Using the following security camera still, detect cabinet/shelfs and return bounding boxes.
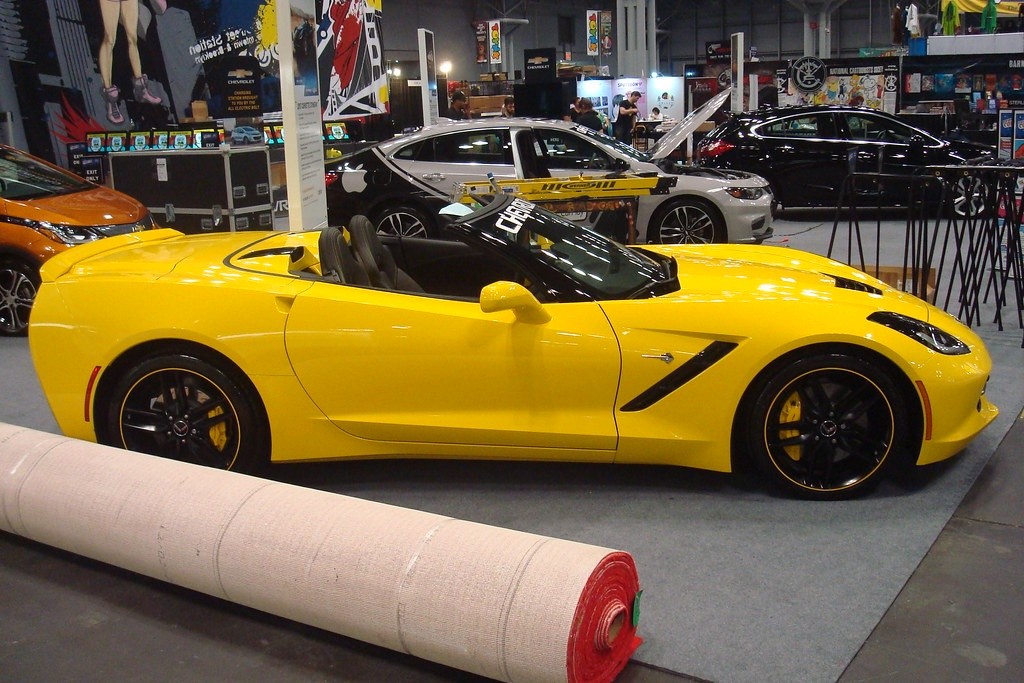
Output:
[897,100,956,115]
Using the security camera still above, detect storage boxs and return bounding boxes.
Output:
[558,65,596,79]
[479,73,493,82]
[849,265,935,309]
[148,207,274,235]
[494,73,506,81]
[463,95,515,119]
[109,145,274,215]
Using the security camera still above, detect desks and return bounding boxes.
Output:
[610,120,664,145]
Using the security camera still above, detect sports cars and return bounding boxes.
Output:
[28,192,999,501]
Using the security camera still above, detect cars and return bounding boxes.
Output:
[325,117,778,245]
[231,126,262,145]
[692,103,998,220]
[0,143,162,337]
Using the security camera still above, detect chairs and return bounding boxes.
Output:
[635,124,648,152]
[318,227,373,288]
[816,113,855,140]
[350,215,426,293]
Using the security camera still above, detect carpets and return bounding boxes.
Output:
[1,215,1023,683]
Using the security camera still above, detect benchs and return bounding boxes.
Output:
[413,138,470,163]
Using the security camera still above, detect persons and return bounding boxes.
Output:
[443,90,474,121]
[495,96,514,118]
[614,90,642,146]
[563,96,604,134]
[647,105,664,122]
